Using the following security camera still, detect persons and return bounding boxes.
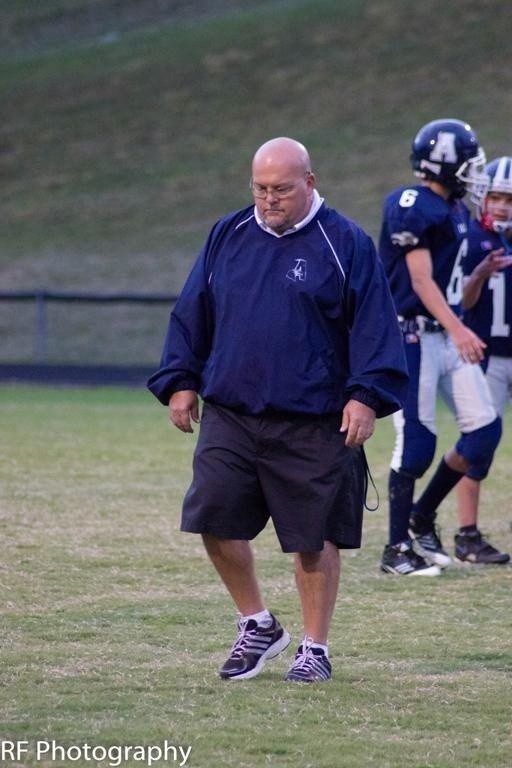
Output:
[145,135,412,686]
[377,118,503,582]
[452,154,511,571]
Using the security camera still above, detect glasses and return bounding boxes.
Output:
[249,171,311,200]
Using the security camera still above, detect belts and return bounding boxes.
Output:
[399,319,444,332]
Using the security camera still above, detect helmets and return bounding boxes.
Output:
[475,157,512,234]
[409,118,490,208]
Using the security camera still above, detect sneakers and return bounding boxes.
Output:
[454,530,510,563]
[284,635,333,683]
[407,503,452,568]
[219,612,292,681]
[380,538,442,576]
